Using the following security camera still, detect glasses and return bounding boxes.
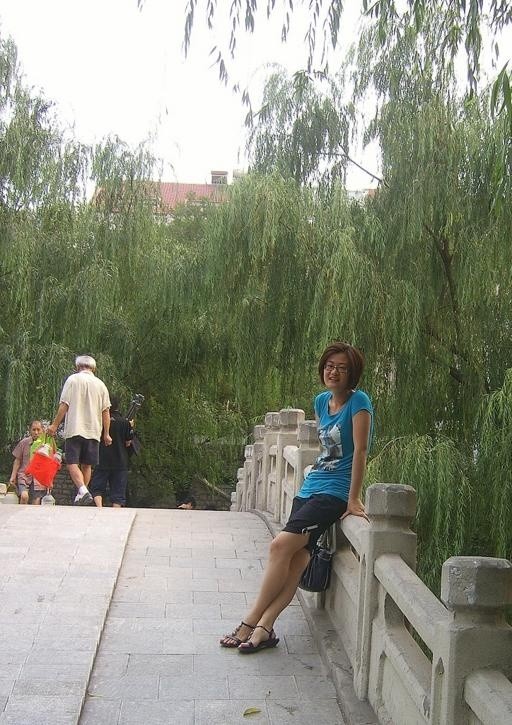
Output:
[324,362,351,375]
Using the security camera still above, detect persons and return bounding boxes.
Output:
[89,396,133,507]
[219,342,374,654]
[9,420,53,505]
[45,355,113,506]
[176,496,196,510]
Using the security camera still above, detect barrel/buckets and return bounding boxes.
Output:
[3,484,19,504]
[41,493,56,506]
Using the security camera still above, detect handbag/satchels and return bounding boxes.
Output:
[298,519,336,593]
[41,488,54,507]
[0,484,19,504]
[24,432,64,486]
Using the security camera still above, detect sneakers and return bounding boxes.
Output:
[74,492,93,506]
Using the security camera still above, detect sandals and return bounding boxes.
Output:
[219,620,253,647]
[236,625,279,652]
